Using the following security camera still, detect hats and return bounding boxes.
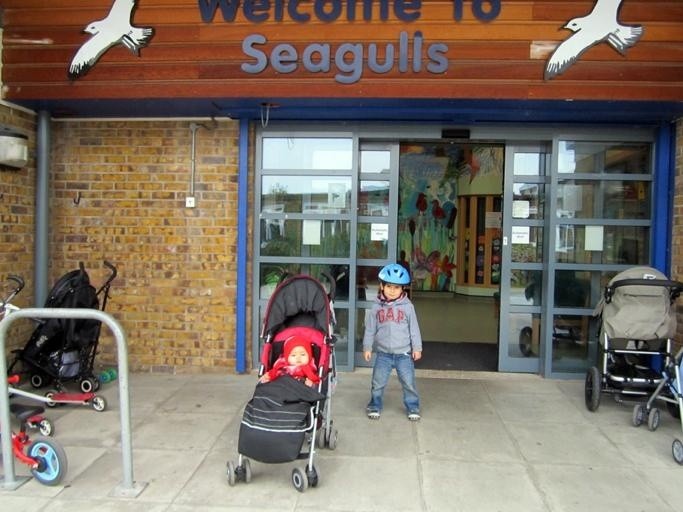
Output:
[284,336,312,363]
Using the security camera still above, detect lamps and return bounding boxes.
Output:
[0,128,28,172]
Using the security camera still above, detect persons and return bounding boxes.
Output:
[258,335,319,387]
[362,264,422,422]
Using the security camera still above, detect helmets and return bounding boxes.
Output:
[378,264,411,286]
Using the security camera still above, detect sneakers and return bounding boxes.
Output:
[407,410,420,420]
[367,411,380,419]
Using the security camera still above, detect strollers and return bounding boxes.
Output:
[584,266,683,468]
[227,270,339,492]
[6,258,117,408]
